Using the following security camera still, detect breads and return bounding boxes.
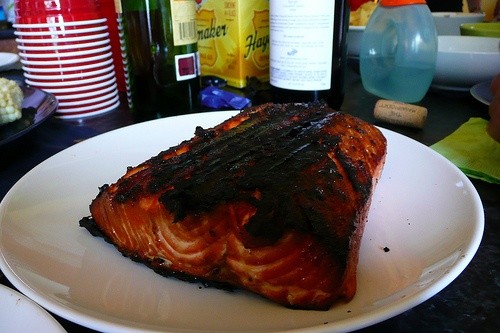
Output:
[0,78,22,124]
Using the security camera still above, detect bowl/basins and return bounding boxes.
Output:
[430,35,500,92]
[430,11,486,36]
[350,25,367,59]
[460,23,500,38]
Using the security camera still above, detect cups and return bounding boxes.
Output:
[13,0,121,121]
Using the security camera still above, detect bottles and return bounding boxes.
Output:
[268,0,349,109]
[123,0,201,120]
[360,0,438,103]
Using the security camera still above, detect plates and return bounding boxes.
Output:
[470,82,492,105]
[0,51,20,71]
[0,110,485,333]
[0,284,67,333]
[0,85,59,145]
